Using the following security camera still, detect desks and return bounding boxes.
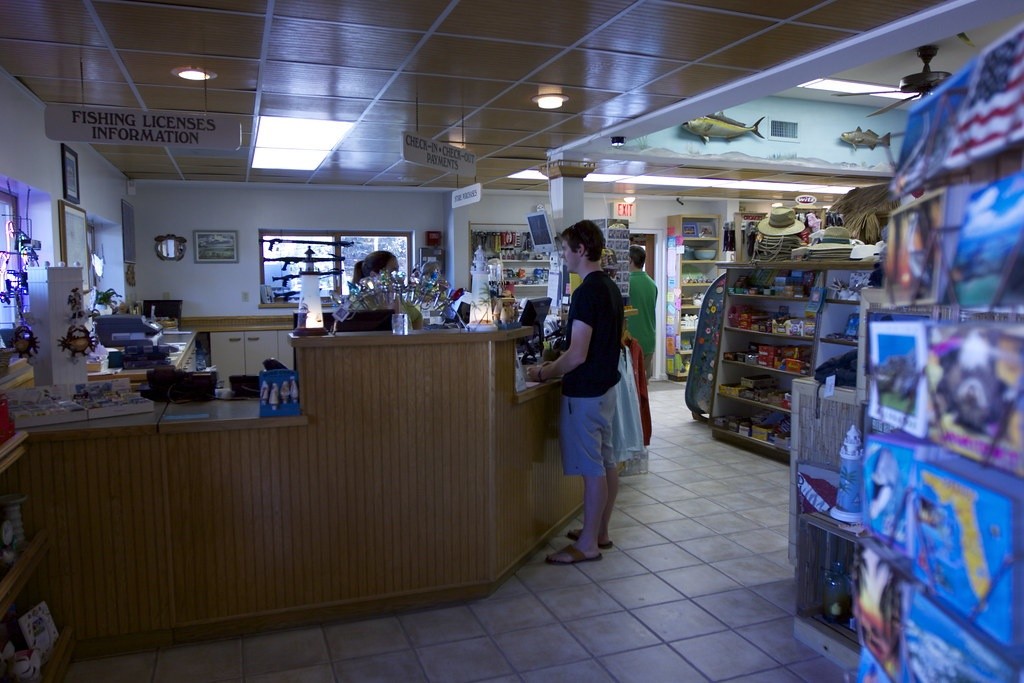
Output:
[17,331,196,658]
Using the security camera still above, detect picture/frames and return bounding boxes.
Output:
[60,142,80,204]
[193,230,239,263]
[58,199,91,293]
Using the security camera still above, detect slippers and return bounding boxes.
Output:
[545,544,602,564]
[566,528,612,548]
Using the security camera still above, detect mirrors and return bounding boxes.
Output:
[155,234,187,261]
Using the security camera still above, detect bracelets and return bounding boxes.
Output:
[538,366,547,383]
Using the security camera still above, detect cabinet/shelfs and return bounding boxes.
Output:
[0,431,78,683]
[709,261,881,464]
[500,259,549,287]
[667,214,723,380]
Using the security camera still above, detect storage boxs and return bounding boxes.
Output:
[740,375,776,388]
[750,424,774,440]
[758,346,781,367]
[738,423,751,438]
[718,383,745,397]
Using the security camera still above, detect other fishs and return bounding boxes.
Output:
[840,126,891,150]
[682,111,766,143]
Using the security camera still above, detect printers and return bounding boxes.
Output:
[94,314,164,354]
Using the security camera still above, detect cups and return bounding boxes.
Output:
[392,313,409,335]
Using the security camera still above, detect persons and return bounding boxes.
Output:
[625,244,659,384]
[332,251,423,334]
[525,219,625,566]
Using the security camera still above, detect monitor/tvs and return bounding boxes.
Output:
[527,211,557,253]
[518,297,552,362]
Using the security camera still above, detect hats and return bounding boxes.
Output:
[750,211,861,261]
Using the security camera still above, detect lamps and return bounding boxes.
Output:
[531,94,569,110]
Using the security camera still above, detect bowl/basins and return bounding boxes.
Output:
[693,249,716,260]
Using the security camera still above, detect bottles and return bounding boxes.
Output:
[821,561,849,623]
[259,376,299,410]
[829,424,864,521]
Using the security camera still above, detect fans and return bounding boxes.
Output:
[832,44,952,118]
